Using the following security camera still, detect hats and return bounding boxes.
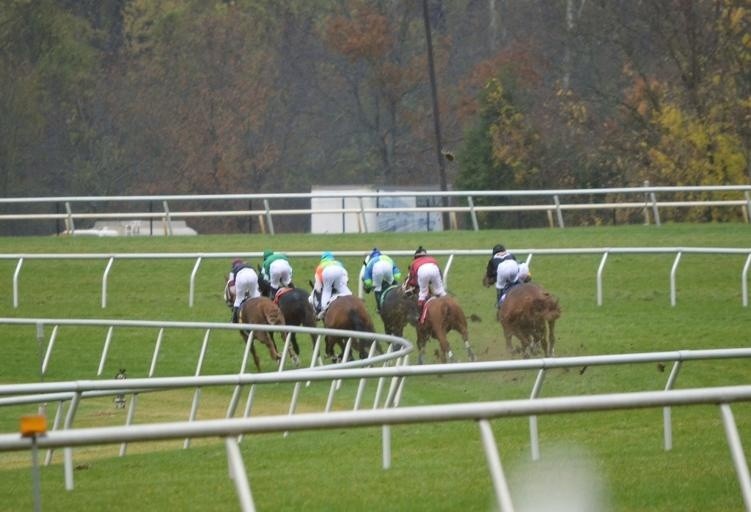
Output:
[263,249,275,261]
[322,252,335,260]
[415,246,428,258]
[493,244,505,255]
[369,248,382,258]
[233,259,245,267]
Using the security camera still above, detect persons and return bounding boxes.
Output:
[404,245,447,313]
[310,253,352,320]
[482,245,531,306]
[260,249,294,301]
[359,248,402,315]
[223,259,262,323]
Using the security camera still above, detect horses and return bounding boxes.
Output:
[483,274,547,358]
[258,263,325,366]
[363,260,422,367]
[541,296,561,357]
[406,264,477,364]
[224,275,300,373]
[309,279,384,365]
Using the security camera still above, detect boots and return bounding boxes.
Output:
[374,289,383,313]
[231,306,238,323]
[287,283,298,287]
[495,288,504,304]
[271,286,278,302]
[416,299,424,322]
